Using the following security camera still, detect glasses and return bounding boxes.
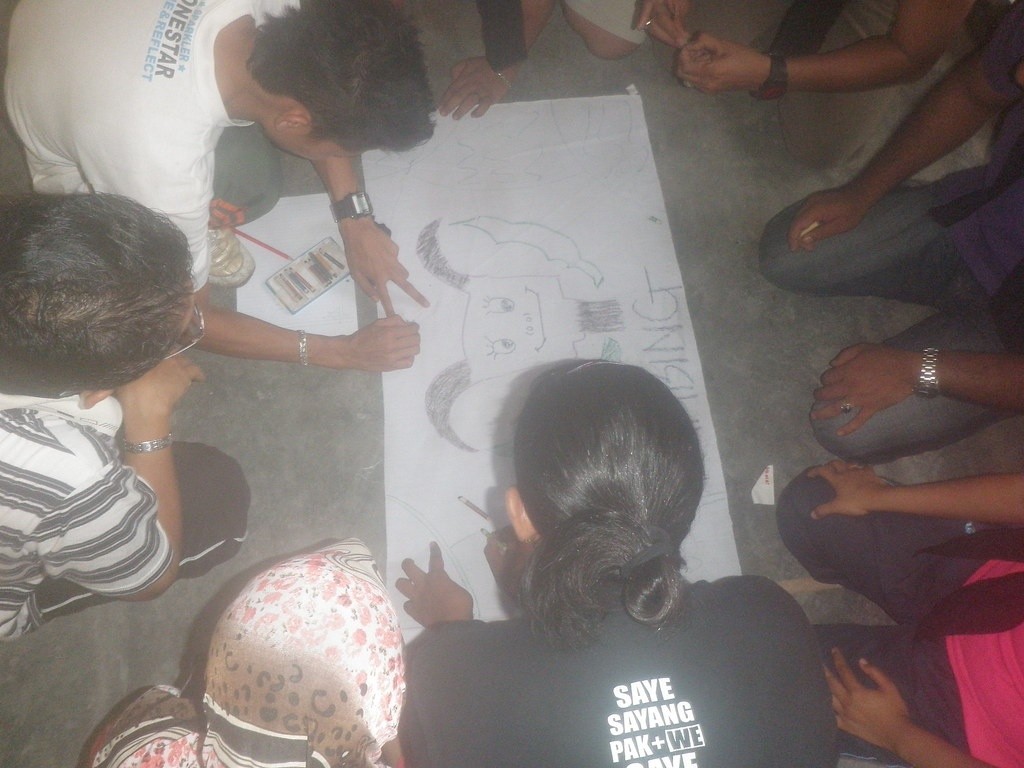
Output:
[60,302,205,401]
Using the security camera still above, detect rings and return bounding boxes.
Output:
[681,81,692,90]
[840,398,853,413]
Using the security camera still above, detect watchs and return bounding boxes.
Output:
[750,50,787,102]
[330,190,373,223]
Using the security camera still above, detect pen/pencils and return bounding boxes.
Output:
[230,226,295,262]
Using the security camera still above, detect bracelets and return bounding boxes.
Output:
[296,330,310,369]
[493,68,513,92]
[119,432,175,454]
[916,344,942,396]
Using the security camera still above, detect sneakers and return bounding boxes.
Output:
[204,227,256,289]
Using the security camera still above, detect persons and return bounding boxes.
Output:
[760,3,1024,464]
[0,189,248,644]
[431,1,657,120]
[778,456,1024,768]
[90,545,406,768]
[632,1,975,102]
[397,355,837,768]
[0,0,433,374]
